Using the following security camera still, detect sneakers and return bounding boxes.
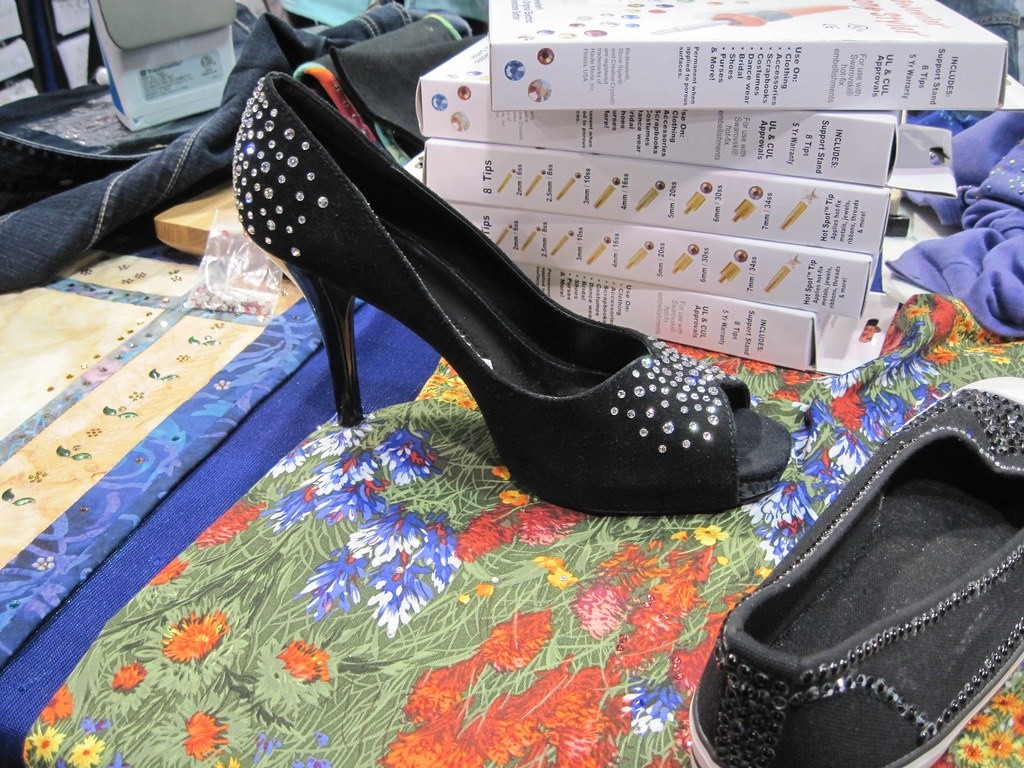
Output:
[681,372,1024,768]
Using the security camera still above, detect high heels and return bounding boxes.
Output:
[228,70,795,518]
